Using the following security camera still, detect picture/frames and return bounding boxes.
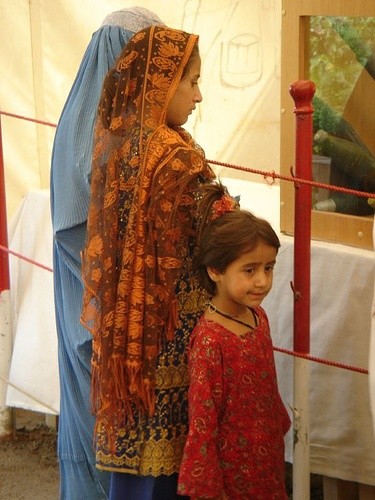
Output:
[281,2,373,251]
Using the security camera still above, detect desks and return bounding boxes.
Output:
[10,178,375,500]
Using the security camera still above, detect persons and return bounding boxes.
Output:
[179,183,291,500]
[48,9,166,500]
[81,25,222,500]
